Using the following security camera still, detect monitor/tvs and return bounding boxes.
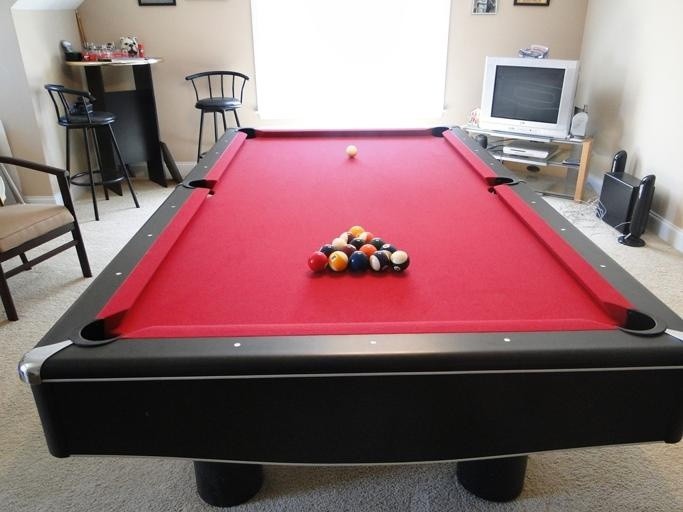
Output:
[479,56,580,139]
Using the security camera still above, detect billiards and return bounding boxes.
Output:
[308,226,409,273]
[347,145,357,156]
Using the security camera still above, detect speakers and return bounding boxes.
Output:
[570,112,590,139]
[618,175,655,246]
[611,151,627,172]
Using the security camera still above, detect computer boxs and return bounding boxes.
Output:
[596,172,642,234]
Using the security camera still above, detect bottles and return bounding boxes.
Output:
[137,43,144,57]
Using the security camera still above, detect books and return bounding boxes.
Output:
[109,57,141,64]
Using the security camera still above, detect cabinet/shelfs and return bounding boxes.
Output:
[462,124,593,203]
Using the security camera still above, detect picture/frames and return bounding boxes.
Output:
[471,0,498,15]
[513,0,551,7]
[137,0,178,7]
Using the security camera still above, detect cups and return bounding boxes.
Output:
[82,42,127,62]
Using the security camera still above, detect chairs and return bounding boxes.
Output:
[1,156,91,323]
[45,83,140,221]
[184,70,249,163]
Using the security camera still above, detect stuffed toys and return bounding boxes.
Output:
[119,37,138,57]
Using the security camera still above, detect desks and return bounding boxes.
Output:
[18,126,683,511]
[66,57,184,187]
